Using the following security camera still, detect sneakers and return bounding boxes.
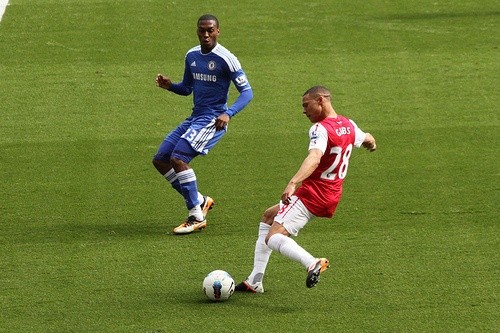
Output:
[173,215,207,235]
[235,280,264,293]
[200,196,215,218]
[305,257,330,288]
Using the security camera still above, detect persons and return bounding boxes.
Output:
[153,14,254,234]
[235,85,376,293]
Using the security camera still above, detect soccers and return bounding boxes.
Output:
[202,269,235,303]
